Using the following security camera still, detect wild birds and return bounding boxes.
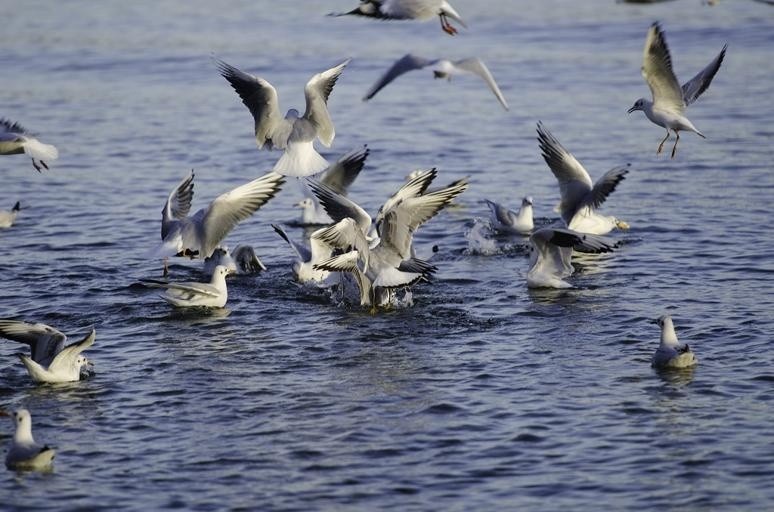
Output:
[0,318,96,384]
[159,265,237,307]
[173,244,267,276]
[360,52,510,113]
[274,144,470,317]
[0,117,58,174]
[484,120,631,290]
[212,56,351,179]
[651,313,696,370]
[0,200,31,229]
[0,408,55,469]
[627,20,728,160]
[326,0,467,36]
[161,169,287,261]
